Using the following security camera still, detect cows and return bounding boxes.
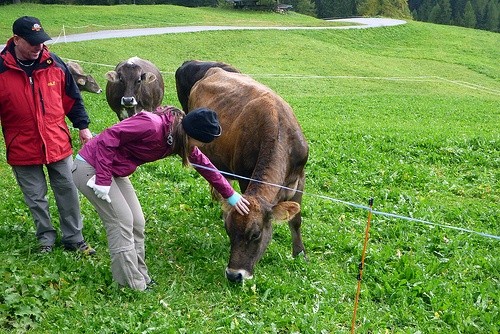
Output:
[174,60,241,116]
[185,66,309,282]
[64,60,102,94]
[105,57,166,123]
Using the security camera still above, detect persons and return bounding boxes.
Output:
[0,16,96,255]
[71,106,250,292]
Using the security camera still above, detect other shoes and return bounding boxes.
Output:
[64,239,96,256]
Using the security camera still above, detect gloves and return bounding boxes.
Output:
[86,175,112,205]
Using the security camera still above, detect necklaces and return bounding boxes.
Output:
[17,58,35,66]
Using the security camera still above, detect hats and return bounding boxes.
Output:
[13,15,50,46]
[181,109,222,144]
